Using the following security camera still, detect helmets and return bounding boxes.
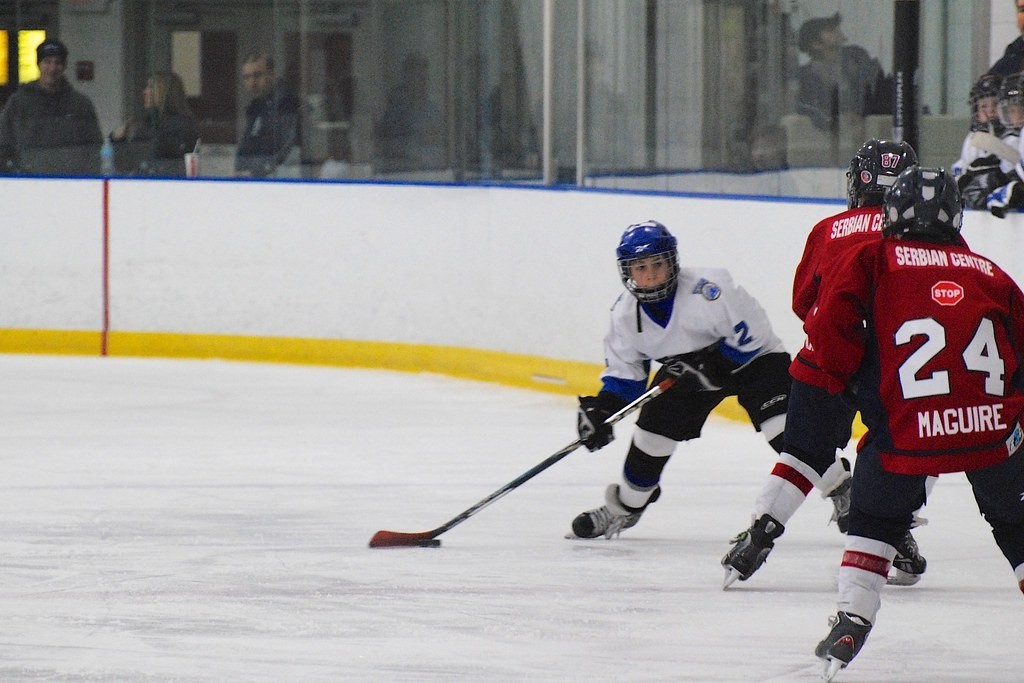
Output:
[848,140,919,207]
[615,221,680,301]
[883,165,962,239]
[970,72,1023,135]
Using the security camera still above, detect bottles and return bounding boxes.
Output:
[101,138,115,177]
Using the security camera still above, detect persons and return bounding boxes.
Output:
[233,54,309,176]
[0,39,103,176]
[814,165,1024,683]
[947,1,1024,219]
[794,11,888,123]
[720,140,927,589]
[565,221,854,542]
[374,54,536,175]
[110,71,199,178]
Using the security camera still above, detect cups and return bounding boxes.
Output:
[184,153,200,177]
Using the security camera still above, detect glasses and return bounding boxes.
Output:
[242,71,275,81]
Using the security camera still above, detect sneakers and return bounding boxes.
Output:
[888,534,928,589]
[823,457,853,533]
[723,515,787,593]
[570,484,662,538]
[815,586,882,680]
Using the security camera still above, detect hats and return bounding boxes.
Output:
[800,12,840,49]
[36,41,68,63]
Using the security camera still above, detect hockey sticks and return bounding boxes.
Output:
[368,375,676,548]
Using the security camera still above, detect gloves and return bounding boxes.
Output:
[987,180,1023,218]
[578,395,616,450]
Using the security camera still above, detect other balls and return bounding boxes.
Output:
[418,539,441,548]
